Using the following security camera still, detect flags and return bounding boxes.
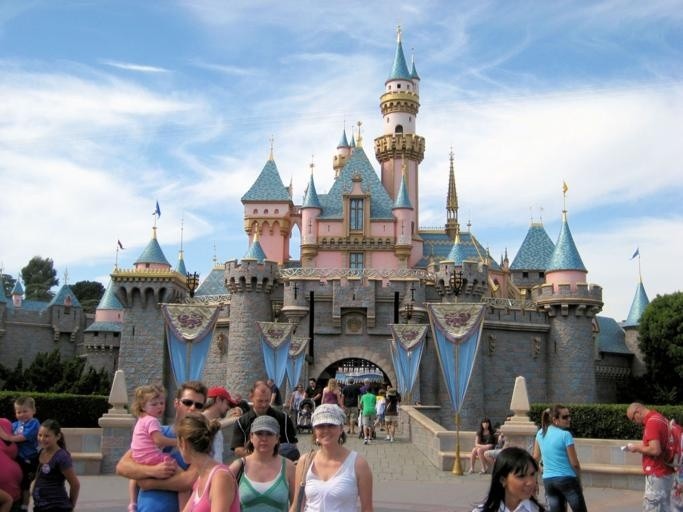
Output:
[562,183,567,192]
[632,249,639,259]
[156,202,161,216]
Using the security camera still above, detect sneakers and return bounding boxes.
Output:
[363,436,374,444]
[385,438,395,442]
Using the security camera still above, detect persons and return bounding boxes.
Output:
[623,402,682,512]
[532,404,588,512]
[127,382,191,512]
[470,446,544,512]
[287,404,373,512]
[203,385,240,463]
[468,416,494,475]
[116,380,209,512]
[268,379,283,411]
[228,415,306,511]
[0,395,80,512]
[286,377,402,444]
[484,430,509,464]
[173,412,241,512]
[230,380,300,461]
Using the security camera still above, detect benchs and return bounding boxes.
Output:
[441,451,646,491]
[70,451,103,475]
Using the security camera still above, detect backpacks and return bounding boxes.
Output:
[645,414,683,466]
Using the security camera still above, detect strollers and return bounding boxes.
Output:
[294,397,316,434]
[356,410,377,439]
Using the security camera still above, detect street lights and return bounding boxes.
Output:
[271,300,284,386]
[402,298,415,404]
[448,268,468,476]
[183,268,202,385]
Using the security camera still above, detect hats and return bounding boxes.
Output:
[311,403,346,428]
[386,388,397,396]
[207,387,239,406]
[250,415,281,435]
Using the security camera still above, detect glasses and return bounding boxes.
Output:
[556,413,572,420]
[178,398,204,410]
